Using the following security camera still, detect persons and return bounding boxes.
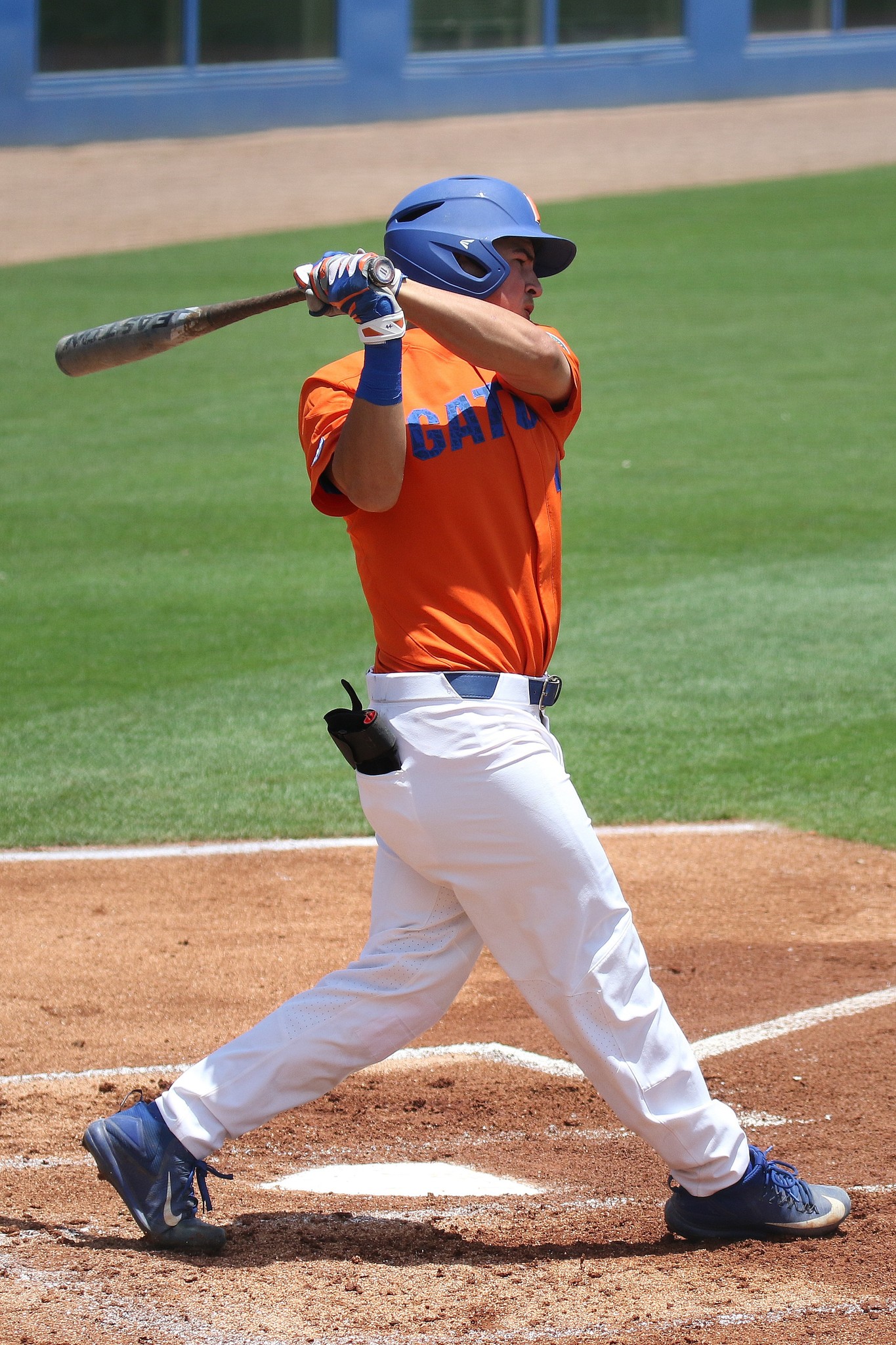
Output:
[84,176,851,1248]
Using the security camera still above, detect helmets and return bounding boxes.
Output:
[383,175,578,299]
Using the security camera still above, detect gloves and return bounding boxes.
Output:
[293,249,408,346]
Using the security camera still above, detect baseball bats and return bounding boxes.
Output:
[53,256,396,378]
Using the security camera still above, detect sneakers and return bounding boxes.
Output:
[664,1144,850,1240]
[82,1090,235,1255]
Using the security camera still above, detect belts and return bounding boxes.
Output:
[444,671,563,711]
